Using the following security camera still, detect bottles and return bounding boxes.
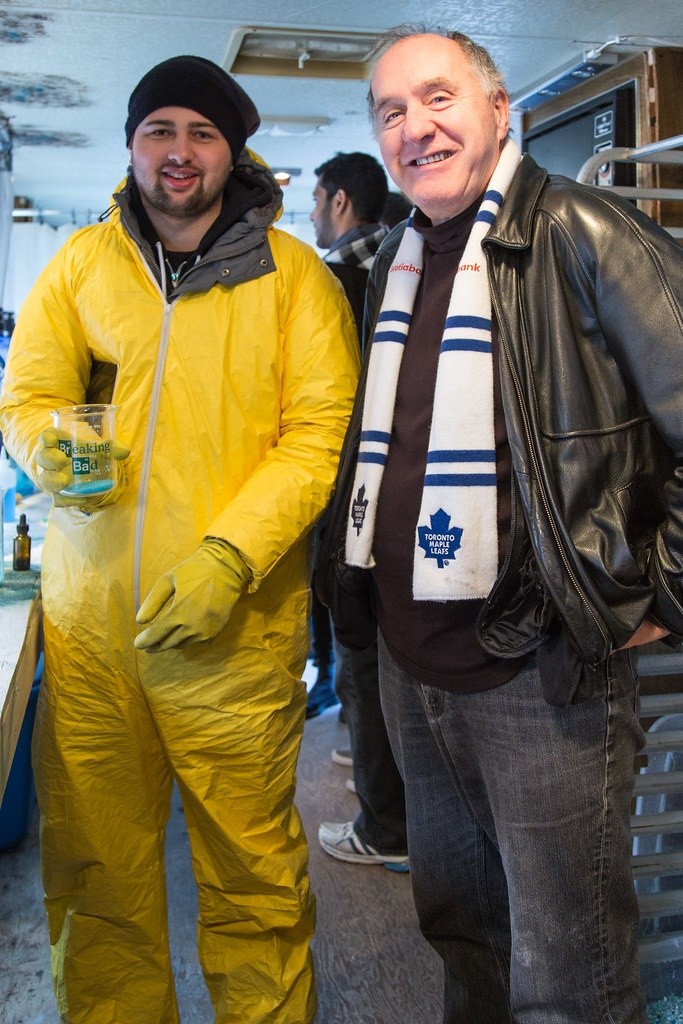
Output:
[13,514,32,571]
[0,446,16,522]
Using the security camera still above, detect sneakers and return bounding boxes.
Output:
[318,822,410,873]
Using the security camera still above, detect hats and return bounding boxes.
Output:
[125,55,261,167]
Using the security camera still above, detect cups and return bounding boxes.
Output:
[51,403,119,498]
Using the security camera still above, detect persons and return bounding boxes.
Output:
[0,52,359,1024]
[380,192,413,230]
[315,26,683,1024]
[306,149,415,873]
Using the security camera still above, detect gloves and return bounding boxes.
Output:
[18,427,130,508]
[134,538,252,654]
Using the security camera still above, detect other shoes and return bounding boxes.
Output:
[305,680,340,717]
[331,746,354,766]
